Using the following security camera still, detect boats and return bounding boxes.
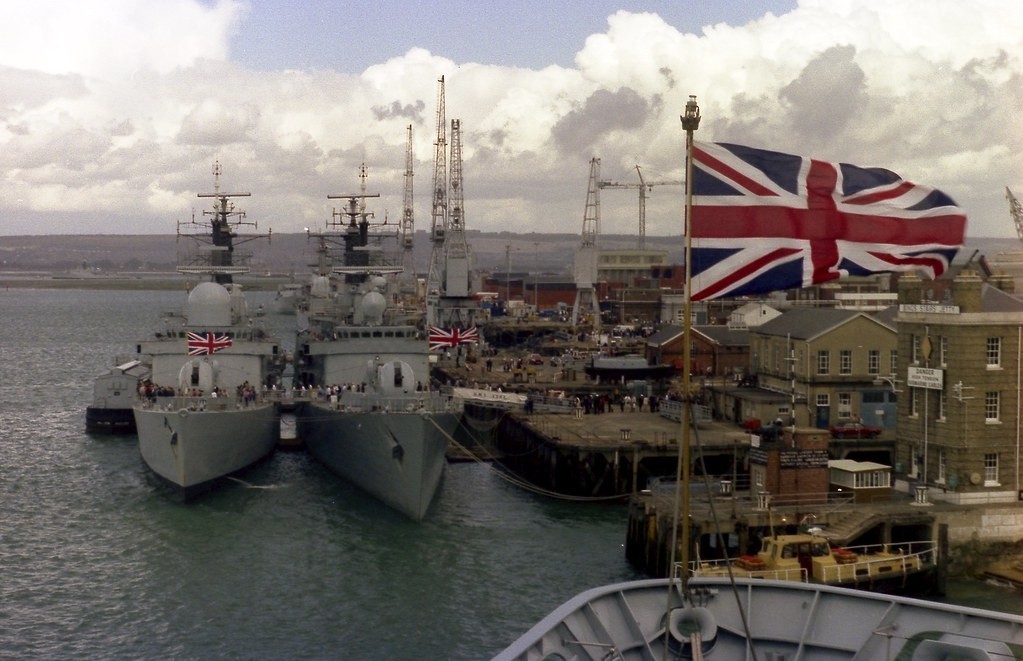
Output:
[85,159,465,524]
[674,533,938,598]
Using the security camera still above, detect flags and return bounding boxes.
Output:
[183,329,234,356]
[684,138,967,304]
[425,325,482,352]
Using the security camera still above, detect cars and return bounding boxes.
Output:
[525,354,544,365]
[830,422,882,440]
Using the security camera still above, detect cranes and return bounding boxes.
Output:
[599,165,686,249]
[571,156,602,327]
[393,76,468,330]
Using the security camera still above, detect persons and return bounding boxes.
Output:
[415,373,703,417]
[137,377,368,409]
